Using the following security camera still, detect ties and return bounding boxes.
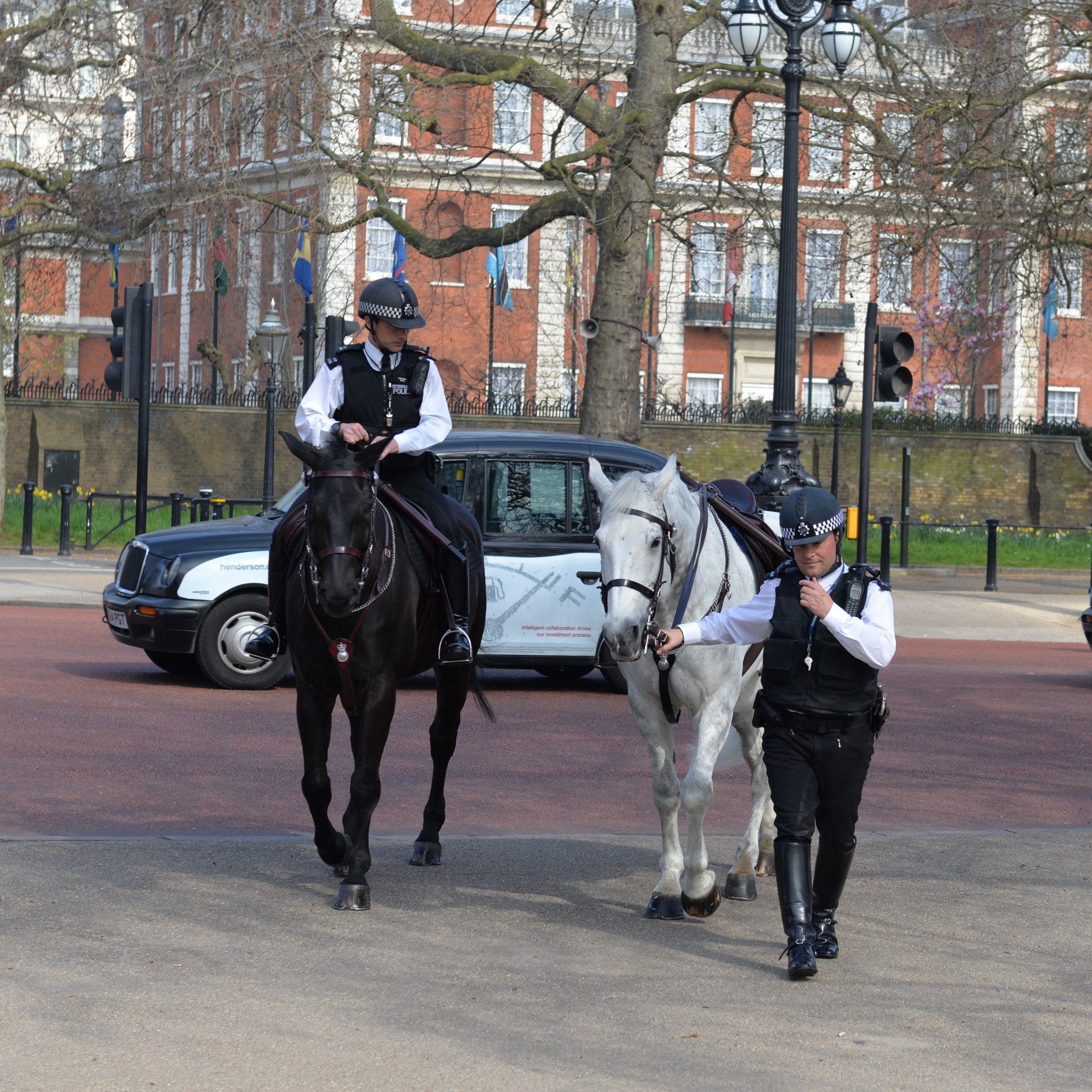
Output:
[381,354,391,374]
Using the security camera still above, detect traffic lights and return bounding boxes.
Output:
[105,286,140,402]
[324,315,359,359]
[873,324,915,405]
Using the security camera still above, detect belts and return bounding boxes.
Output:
[785,712,868,733]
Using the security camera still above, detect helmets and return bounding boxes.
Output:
[779,487,844,545]
[357,277,426,329]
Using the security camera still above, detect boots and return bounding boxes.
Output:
[812,833,857,958]
[774,842,819,978]
[245,544,291,657]
[429,540,471,666]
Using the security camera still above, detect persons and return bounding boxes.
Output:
[658,486,897,980]
[294,275,474,660]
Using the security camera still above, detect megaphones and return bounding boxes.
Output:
[578,319,599,339]
[642,335,662,352]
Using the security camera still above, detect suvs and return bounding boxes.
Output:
[99,425,696,695]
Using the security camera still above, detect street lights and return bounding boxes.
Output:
[724,0,863,512]
[827,358,854,501]
[255,296,293,511]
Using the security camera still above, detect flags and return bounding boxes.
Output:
[3,215,738,326]
[1040,276,1061,341]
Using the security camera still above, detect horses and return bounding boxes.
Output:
[582,453,782,921]
[261,427,485,912]
